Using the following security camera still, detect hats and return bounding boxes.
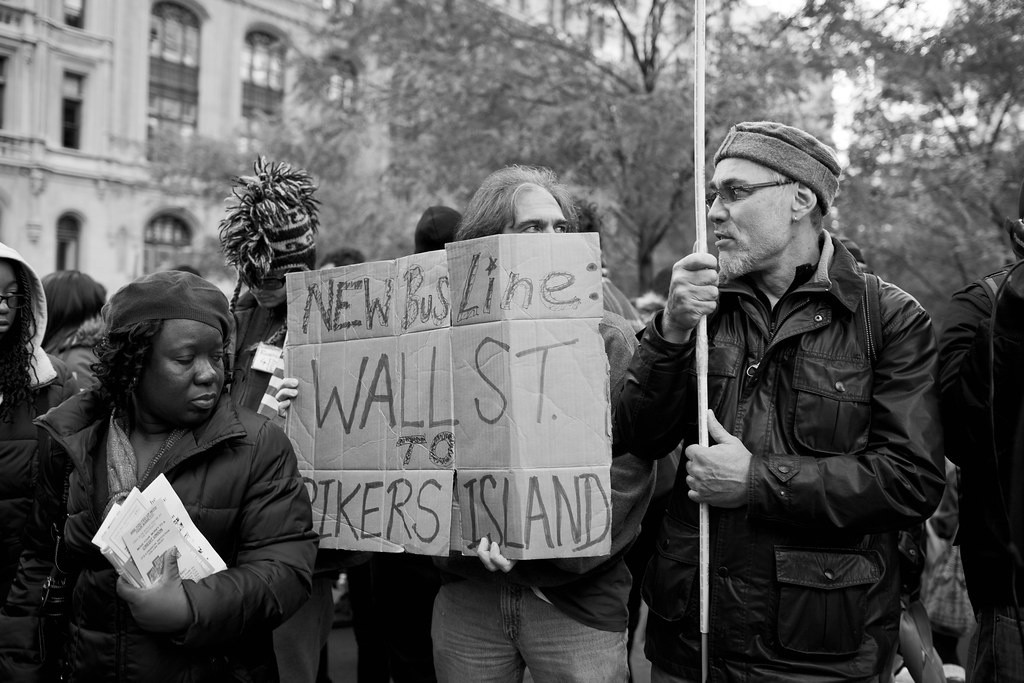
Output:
[217,153,323,352]
[101,269,235,348]
[712,120,842,217]
[413,206,463,254]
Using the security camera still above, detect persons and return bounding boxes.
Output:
[619,120,945,683]
[1,254,319,682]
[412,205,463,253]
[920,456,975,683]
[277,166,655,683]
[39,268,110,393]
[919,176,1023,683]
[220,191,333,681]
[628,266,675,326]
[568,191,649,346]
[319,246,364,269]
[0,240,78,598]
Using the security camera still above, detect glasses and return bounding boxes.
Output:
[253,268,293,291]
[705,179,796,212]
[0,294,30,310]
